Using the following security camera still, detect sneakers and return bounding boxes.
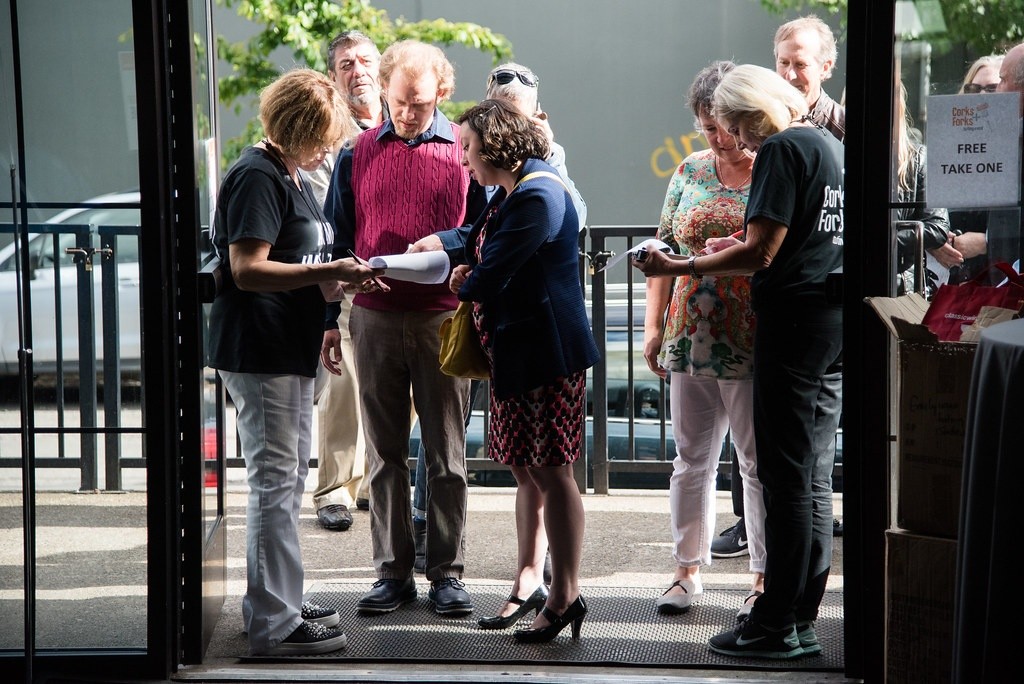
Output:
[708,618,804,658]
[833,518,843,536]
[711,518,749,557]
[301,601,340,627]
[798,620,822,653]
[255,621,347,656]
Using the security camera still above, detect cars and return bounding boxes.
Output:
[0,187,214,389]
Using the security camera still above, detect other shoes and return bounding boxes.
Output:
[318,505,354,528]
[414,566,426,572]
[736,591,762,621]
[544,552,552,582]
[356,498,369,509]
[657,578,703,614]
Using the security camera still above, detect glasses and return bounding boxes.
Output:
[486,69,540,94]
[963,83,999,94]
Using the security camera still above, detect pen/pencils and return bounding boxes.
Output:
[347,248,385,294]
[697,229,744,254]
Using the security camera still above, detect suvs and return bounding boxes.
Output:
[406,282,844,492]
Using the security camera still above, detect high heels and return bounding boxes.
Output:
[513,594,587,643]
[478,583,549,628]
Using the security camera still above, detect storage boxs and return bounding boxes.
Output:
[864,289,1019,540]
[883,527,957,684]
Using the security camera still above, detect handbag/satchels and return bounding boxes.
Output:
[438,301,489,380]
[921,262,1024,341]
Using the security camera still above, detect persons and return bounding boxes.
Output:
[627,64,844,660]
[414,62,586,587]
[320,40,487,614]
[895,44,1024,301]
[213,27,391,656]
[643,64,766,622]
[314,31,391,530]
[449,99,600,643]
[711,18,842,558]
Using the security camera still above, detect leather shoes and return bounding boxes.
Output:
[356,576,417,611]
[429,578,474,613]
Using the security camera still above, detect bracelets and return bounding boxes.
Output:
[687,256,703,281]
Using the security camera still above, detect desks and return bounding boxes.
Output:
[953,318,1024,684]
